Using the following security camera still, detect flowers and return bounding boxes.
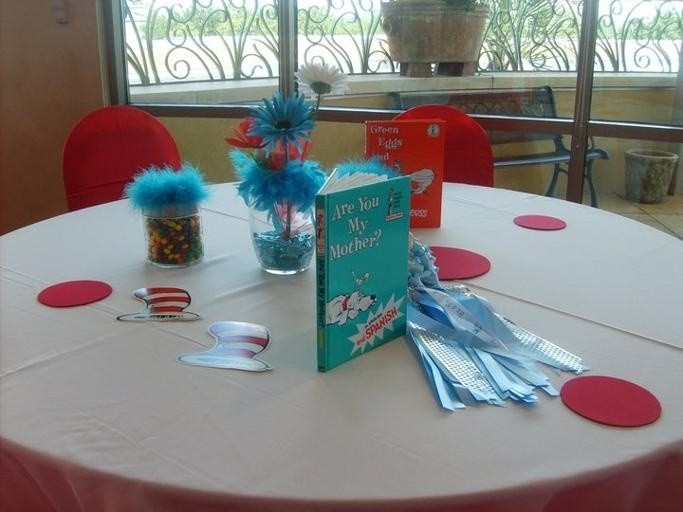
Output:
[224,62,353,238]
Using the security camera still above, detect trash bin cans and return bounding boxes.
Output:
[624,149,679,204]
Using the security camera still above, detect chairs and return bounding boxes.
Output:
[390,102,496,188]
[62,104,182,212]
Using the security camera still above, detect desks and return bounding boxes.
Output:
[0,172,682,511]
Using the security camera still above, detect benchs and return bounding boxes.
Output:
[391,85,609,208]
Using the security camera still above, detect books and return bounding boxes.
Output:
[363,119,448,228]
[310,163,418,374]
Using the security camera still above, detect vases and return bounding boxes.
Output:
[244,203,319,276]
